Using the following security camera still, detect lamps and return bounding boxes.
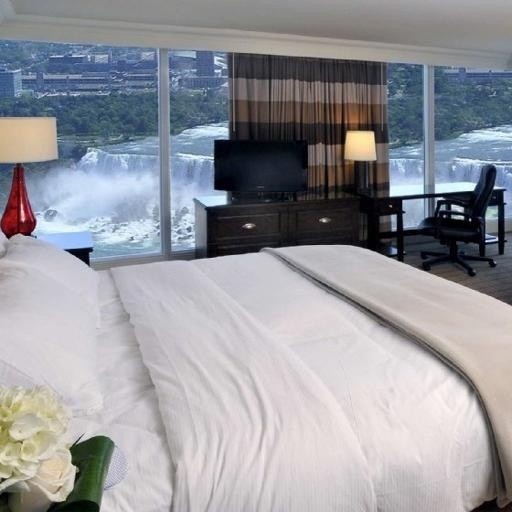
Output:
[0,117,59,239]
[344,130,377,194]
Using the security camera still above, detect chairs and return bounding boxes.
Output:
[421,165,498,277]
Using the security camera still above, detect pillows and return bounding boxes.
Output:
[0,265,103,416]
[0,232,104,325]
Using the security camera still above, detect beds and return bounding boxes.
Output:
[0,228,512,512]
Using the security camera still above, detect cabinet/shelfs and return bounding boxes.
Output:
[192,196,359,260]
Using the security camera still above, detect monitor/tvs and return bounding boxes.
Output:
[212,137,309,204]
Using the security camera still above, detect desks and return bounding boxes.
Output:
[355,181,507,264]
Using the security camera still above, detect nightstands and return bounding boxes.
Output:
[30,234,94,267]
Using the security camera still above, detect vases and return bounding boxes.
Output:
[8,487,51,512]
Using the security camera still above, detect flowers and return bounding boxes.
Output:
[0,383,77,502]
